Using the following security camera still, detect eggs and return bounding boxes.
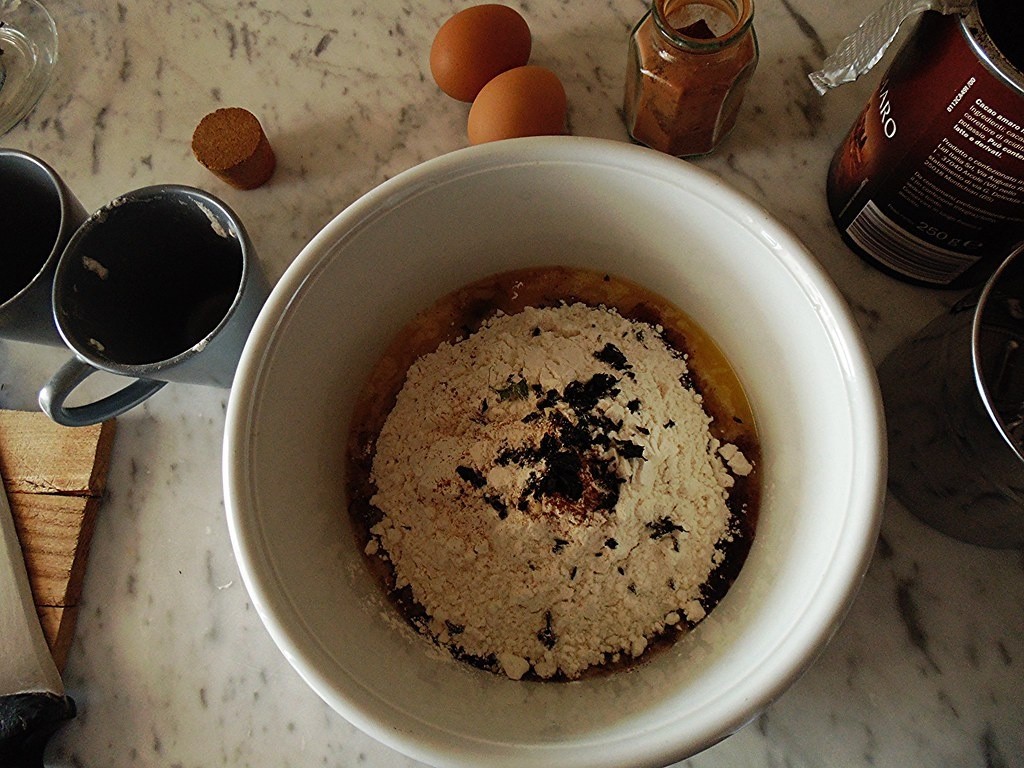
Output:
[431,4,532,103]
[466,65,568,145]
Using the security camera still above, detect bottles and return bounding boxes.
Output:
[620,0,759,159]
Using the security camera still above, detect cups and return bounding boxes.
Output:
[222,137,888,768]
[827,0,1024,552]
[0,147,267,429]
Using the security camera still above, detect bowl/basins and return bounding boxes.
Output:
[0,0,62,143]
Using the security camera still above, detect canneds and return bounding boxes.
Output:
[827,0,1024,549]
